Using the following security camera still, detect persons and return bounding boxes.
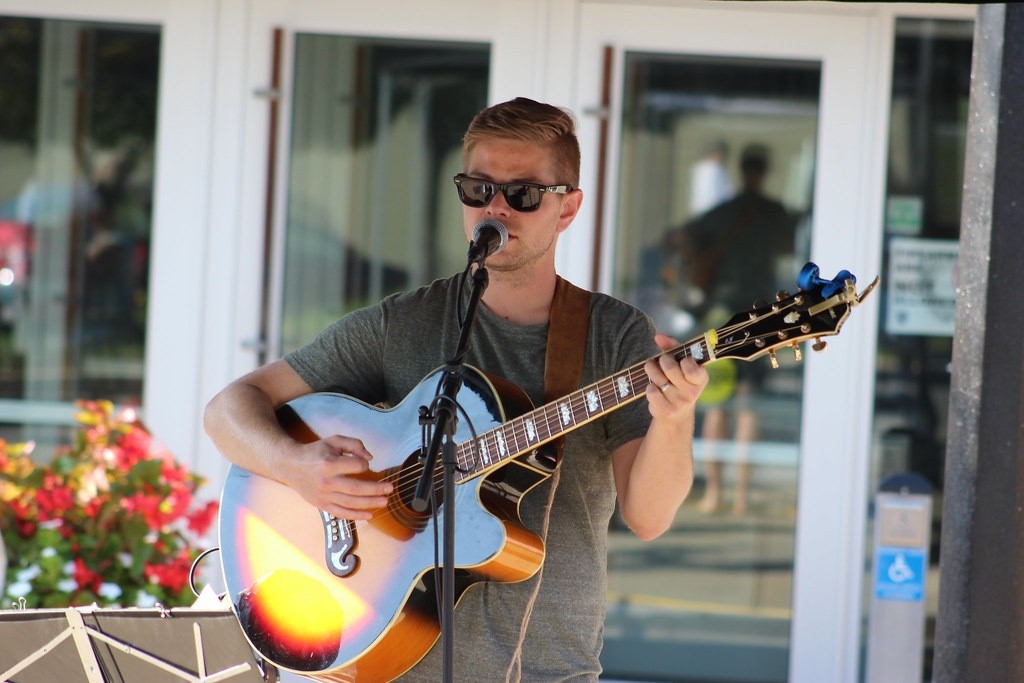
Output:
[689,142,807,512]
[682,138,733,321]
[7,105,148,361]
[204,98,709,683]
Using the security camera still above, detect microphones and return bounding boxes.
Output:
[467,219,509,263]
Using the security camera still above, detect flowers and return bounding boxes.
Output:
[1,395,217,614]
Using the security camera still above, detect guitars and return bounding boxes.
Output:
[217,261,881,683]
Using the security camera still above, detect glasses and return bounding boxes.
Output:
[453,172,570,213]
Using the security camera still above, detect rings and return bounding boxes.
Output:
[661,381,672,392]
